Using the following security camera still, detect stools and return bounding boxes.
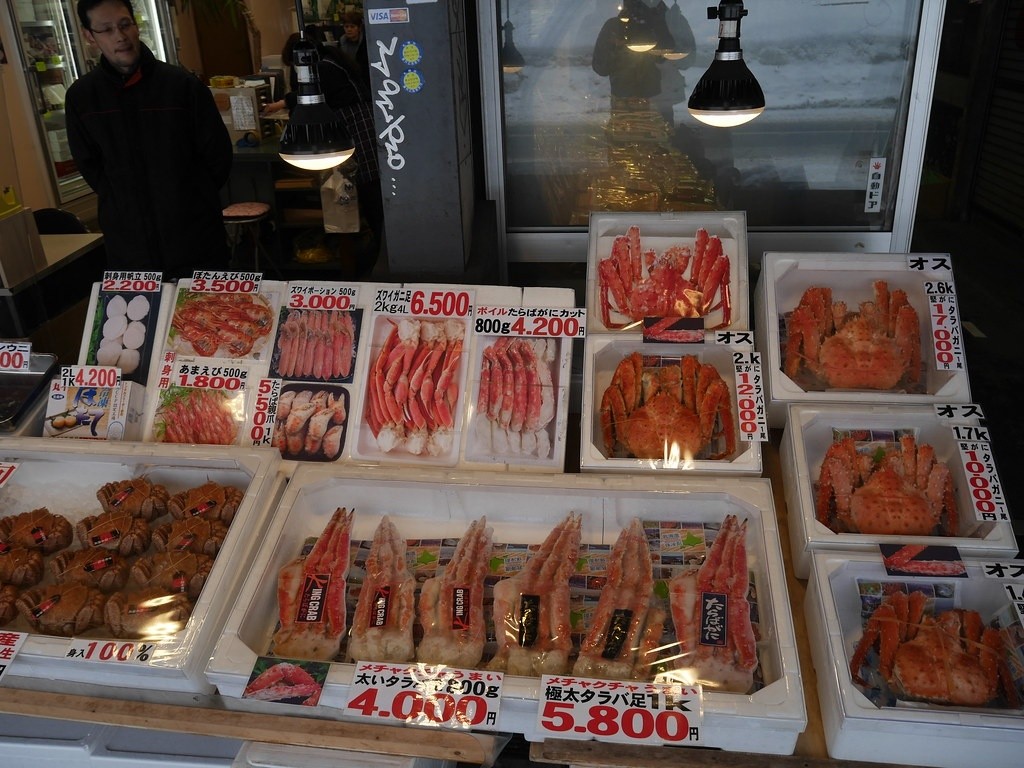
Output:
[223,203,272,283]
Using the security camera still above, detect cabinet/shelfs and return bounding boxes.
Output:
[0,1,177,224]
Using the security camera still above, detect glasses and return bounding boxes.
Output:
[86,19,137,35]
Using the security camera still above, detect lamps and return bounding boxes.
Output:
[498,1,522,74]
[278,0,354,170]
[686,0,766,128]
[653,0,693,60]
[620,1,658,52]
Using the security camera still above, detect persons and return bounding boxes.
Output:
[261,32,384,256]
[305,25,339,47]
[64,0,237,285]
[591,0,696,127]
[337,14,371,61]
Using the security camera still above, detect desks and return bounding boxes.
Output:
[0,235,105,340]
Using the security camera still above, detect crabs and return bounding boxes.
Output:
[0,224,1023,705]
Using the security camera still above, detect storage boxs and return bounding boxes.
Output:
[752,252,971,428]
[0,433,279,708]
[803,551,1024,766]
[206,460,807,756]
[586,210,750,333]
[582,334,763,474]
[778,402,1019,578]
